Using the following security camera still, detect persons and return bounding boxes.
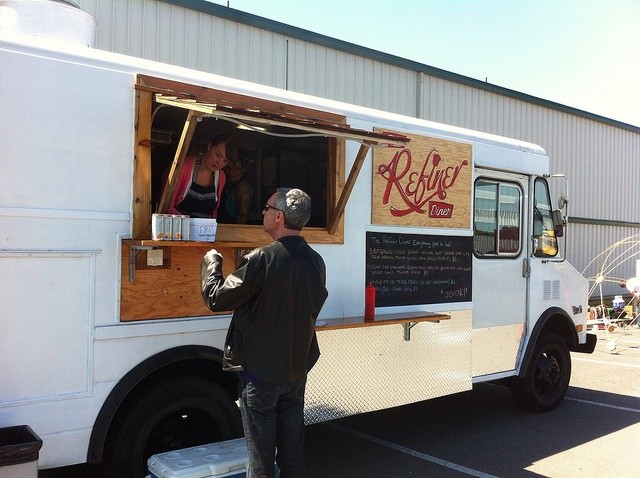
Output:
[168,133,240,219]
[202,189,326,478]
[218,156,253,224]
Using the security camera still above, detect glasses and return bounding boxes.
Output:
[265,204,279,211]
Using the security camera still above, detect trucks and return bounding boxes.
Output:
[0,32,598,478]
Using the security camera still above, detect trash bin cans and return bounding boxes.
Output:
[0,425,43,478]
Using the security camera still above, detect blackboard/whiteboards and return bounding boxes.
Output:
[366,231,472,307]
